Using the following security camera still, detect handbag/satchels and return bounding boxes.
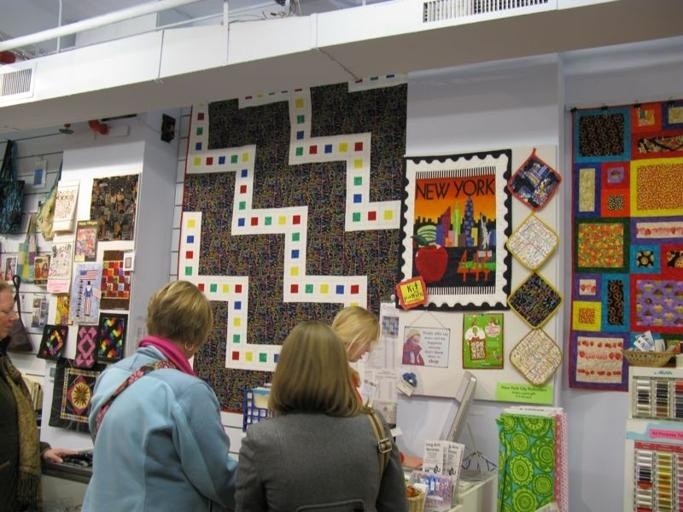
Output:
[0,138,26,234]
[33,161,63,240]
[7,275,33,353]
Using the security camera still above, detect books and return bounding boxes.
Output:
[420,439,467,496]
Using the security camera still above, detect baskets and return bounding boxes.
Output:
[406,487,425,511]
[620,350,678,368]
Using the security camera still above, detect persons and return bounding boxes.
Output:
[79,280,240,511]
[330,304,381,364]
[0,273,79,511]
[230,318,411,512]
[401,328,425,367]
